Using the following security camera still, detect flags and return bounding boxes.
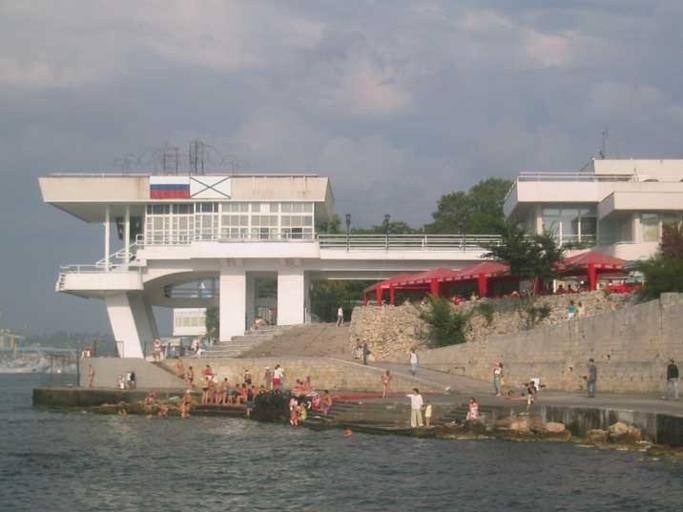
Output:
[190,175,231,199]
[148,175,190,200]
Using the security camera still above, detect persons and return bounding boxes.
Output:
[586,358,596,398]
[576,300,585,317]
[76,315,333,429]
[380,369,392,398]
[335,304,344,327]
[465,396,479,420]
[354,338,362,360]
[661,358,680,400]
[422,399,432,427]
[566,299,576,319]
[493,362,538,410]
[404,387,425,430]
[402,277,614,306]
[342,424,353,439]
[362,340,370,365]
[409,347,420,375]
